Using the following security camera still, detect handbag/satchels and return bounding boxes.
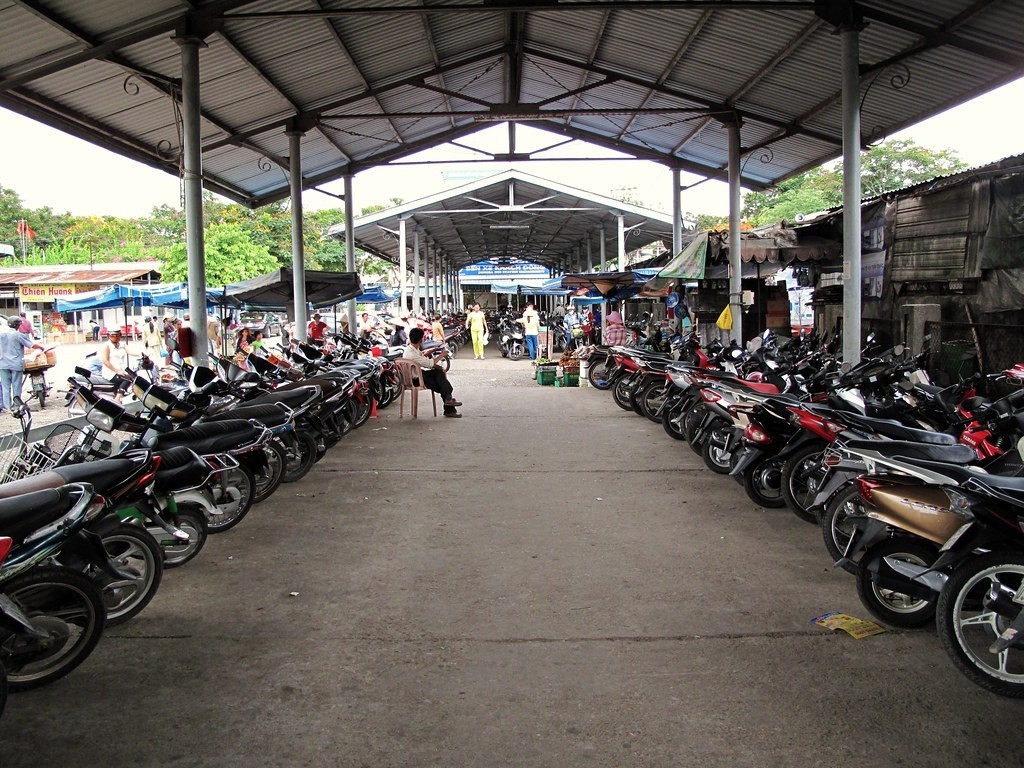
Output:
[160,347,168,357]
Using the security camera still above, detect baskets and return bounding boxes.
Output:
[43,423,112,467]
[0,432,57,486]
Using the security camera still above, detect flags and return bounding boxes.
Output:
[17,221,35,242]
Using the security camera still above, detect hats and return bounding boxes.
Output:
[607,311,623,323]
[337,314,348,323]
[311,314,322,319]
[388,317,406,327]
[567,305,575,310]
[163,312,175,318]
[183,313,189,320]
[144,316,151,322]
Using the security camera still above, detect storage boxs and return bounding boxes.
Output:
[532,362,580,387]
[24,349,55,367]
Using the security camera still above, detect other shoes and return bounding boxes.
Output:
[444,399,462,406]
[446,411,462,418]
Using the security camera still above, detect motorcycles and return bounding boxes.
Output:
[0,305,498,720]
[494,303,1023,699]
[496,309,526,361]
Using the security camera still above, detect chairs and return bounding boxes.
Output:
[394,358,437,420]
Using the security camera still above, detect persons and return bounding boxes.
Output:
[101,329,140,404]
[554,304,570,315]
[234,328,265,355]
[19,312,34,338]
[466,302,489,359]
[403,328,463,418]
[208,311,216,320]
[307,314,331,340]
[143,316,163,360]
[603,311,627,346]
[163,313,191,364]
[631,319,666,349]
[523,302,540,359]
[563,306,601,351]
[90,320,100,341]
[432,314,444,344]
[360,313,375,339]
[0,317,45,415]
[216,318,236,356]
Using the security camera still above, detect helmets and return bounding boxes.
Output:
[7,316,22,327]
[107,323,121,333]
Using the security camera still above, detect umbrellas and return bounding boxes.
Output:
[491,269,663,315]
[53,282,188,367]
[150,267,399,357]
